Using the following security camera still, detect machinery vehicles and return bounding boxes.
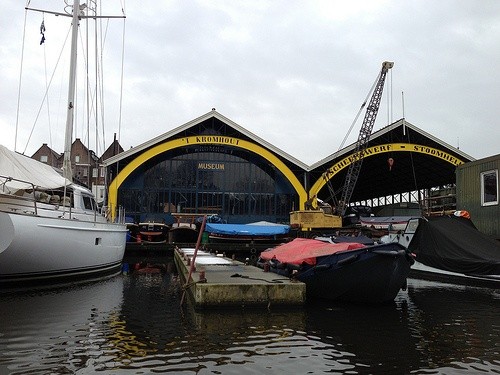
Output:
[290,61,394,235]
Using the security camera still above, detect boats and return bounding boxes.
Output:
[257,237,417,317]
[1,0,129,292]
